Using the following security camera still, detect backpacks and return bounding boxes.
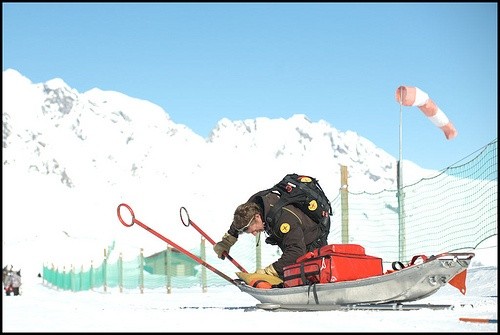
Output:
[251,173,333,254]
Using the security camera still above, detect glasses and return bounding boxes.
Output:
[234,216,255,234]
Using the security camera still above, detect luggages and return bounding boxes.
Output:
[283,244,383,305]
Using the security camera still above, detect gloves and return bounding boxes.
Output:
[254,263,278,277]
[214,233,237,260]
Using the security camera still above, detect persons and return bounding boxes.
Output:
[213,174,332,277]
